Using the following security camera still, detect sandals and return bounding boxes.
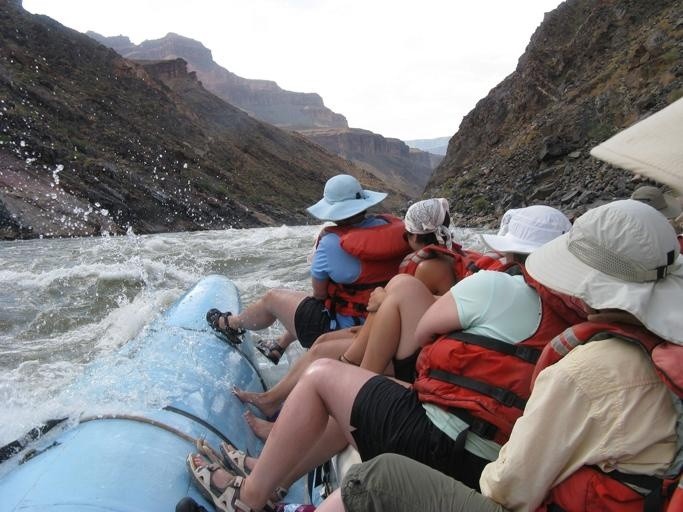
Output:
[219,442,289,511]
[207,309,246,344]
[186,453,253,512]
[255,338,285,365]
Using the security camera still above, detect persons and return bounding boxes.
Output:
[185,97,683,511]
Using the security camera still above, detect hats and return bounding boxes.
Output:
[631,185,683,220]
[306,174,389,222]
[480,205,573,255]
[524,199,683,347]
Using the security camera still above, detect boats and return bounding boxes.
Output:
[0,270,333,510]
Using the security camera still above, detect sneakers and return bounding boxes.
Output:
[176,497,210,512]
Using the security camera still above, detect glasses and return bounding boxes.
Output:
[403,231,412,241]
